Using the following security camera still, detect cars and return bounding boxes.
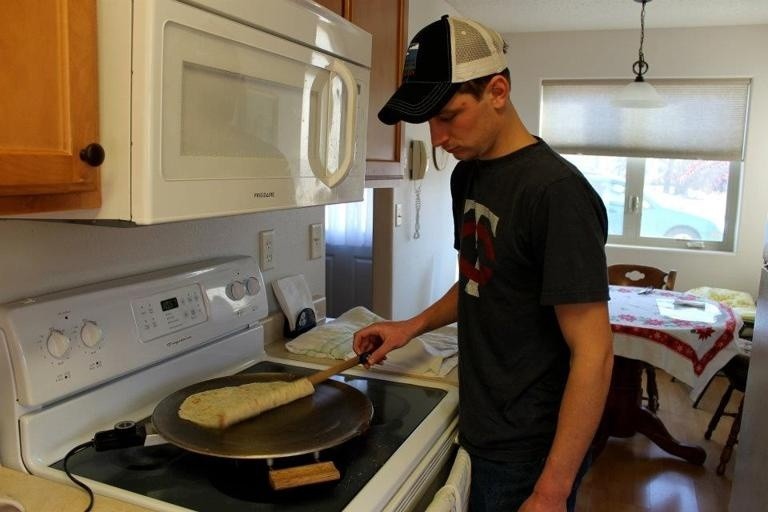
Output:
[585,177,721,242]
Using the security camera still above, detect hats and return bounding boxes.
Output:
[378,14,508,125]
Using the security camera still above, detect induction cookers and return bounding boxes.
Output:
[47,358,449,512]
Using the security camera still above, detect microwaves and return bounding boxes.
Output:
[0,0,374,229]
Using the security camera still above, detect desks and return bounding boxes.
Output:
[592,284,743,465]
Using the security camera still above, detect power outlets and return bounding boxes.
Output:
[259,229,276,271]
[310,223,324,259]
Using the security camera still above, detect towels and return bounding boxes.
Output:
[285,304,459,377]
[424,447,472,511]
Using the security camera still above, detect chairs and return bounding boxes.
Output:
[604,264,676,411]
[692,324,755,477]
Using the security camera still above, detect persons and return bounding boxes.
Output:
[350,12,618,512]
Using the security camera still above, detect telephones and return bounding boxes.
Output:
[409,140,427,180]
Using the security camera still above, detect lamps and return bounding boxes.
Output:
[612,0,666,110]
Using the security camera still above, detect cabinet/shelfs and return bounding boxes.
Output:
[315,0,406,190]
[0,0,106,219]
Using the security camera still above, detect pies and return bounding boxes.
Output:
[180,379,314,429]
